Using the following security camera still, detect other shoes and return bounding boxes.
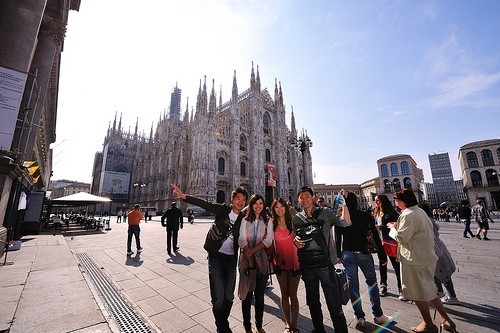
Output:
[440,321,457,333]
[244,328,253,333]
[127,251,134,256]
[412,324,438,333]
[137,246,143,252]
[398,292,407,301]
[483,237,490,240]
[471,234,475,237]
[373,315,393,323]
[355,318,365,329]
[379,282,387,295]
[168,247,179,254]
[476,234,481,240]
[439,294,460,304]
[255,326,266,333]
[463,234,470,238]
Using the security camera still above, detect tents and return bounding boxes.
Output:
[46,192,112,230]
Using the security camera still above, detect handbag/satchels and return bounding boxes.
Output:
[334,192,352,227]
[207,222,225,242]
[263,217,275,255]
[381,240,397,257]
[365,213,379,254]
[239,251,258,276]
[334,268,351,305]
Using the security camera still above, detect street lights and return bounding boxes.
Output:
[133,179,146,206]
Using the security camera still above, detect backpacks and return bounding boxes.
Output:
[294,205,331,270]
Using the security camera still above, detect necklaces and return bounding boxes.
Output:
[278,222,285,227]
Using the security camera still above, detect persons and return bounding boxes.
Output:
[263,196,302,333]
[289,186,353,333]
[431,205,461,223]
[387,188,458,333]
[161,202,184,255]
[417,202,460,305]
[170,182,248,333]
[369,195,408,301]
[317,197,333,210]
[472,199,494,240]
[117,210,127,224]
[334,192,395,326]
[144,209,148,222]
[127,204,143,255]
[459,202,475,239]
[237,193,274,333]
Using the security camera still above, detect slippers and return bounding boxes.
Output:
[284,326,300,333]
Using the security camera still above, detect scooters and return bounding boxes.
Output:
[188,212,195,224]
